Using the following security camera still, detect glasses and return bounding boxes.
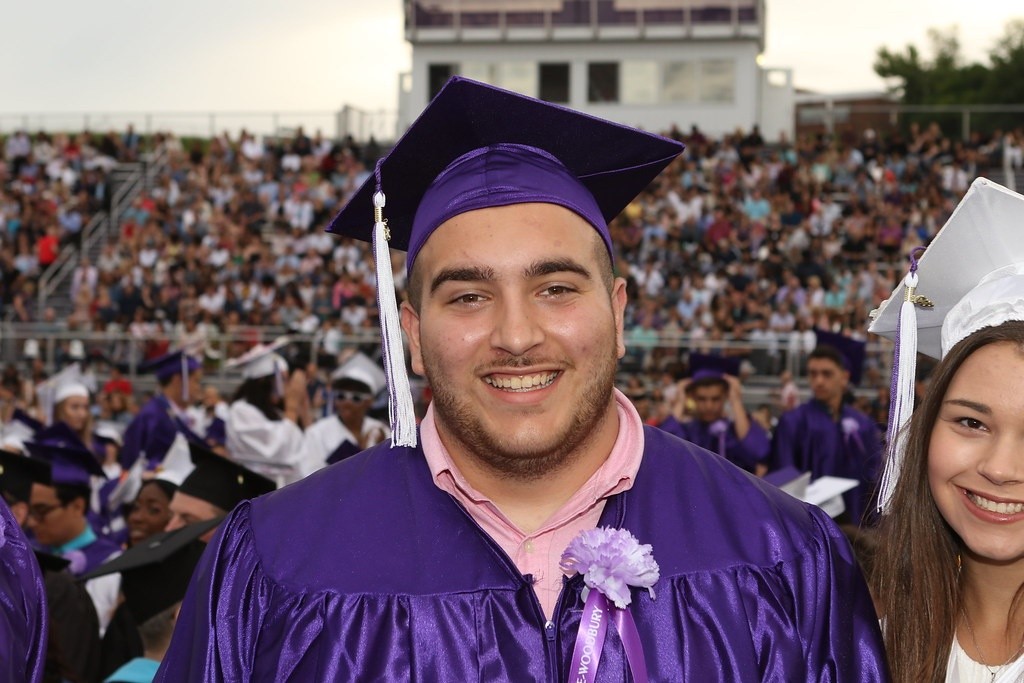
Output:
[26,501,68,523]
[329,389,371,406]
[809,367,842,379]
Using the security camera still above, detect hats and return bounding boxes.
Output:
[868,176,1024,514]
[807,325,866,388]
[324,438,360,464]
[324,76,687,449]
[685,369,729,387]
[2,335,292,506]
[330,352,389,394]
[72,514,230,624]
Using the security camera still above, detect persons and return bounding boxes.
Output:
[0,122,1024,683]
[153,76,893,683]
[868,177,1024,683]
